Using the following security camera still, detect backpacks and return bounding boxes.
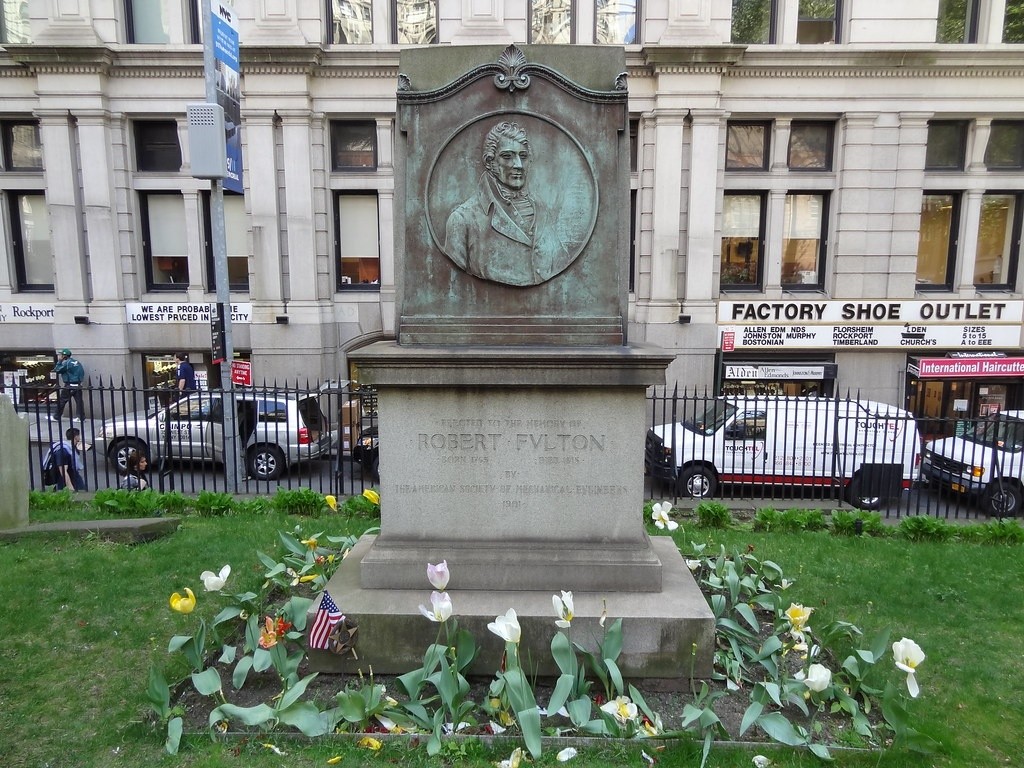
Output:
[45,452,60,486]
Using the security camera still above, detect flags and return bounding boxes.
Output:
[308,589,347,649]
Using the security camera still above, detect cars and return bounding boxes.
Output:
[93,379,351,480]
[352,426,380,483]
[923,409,1024,518]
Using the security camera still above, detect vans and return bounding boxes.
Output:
[645,395,924,512]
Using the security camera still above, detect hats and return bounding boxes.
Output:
[58,349,71,356]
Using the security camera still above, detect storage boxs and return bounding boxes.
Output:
[342,422,360,439]
[342,400,360,425]
[342,431,359,451]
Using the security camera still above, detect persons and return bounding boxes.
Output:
[43,427,85,491]
[43,350,86,423]
[174,353,196,399]
[442,118,572,286]
[120,450,149,491]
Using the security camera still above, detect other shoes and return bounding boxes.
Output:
[45,416,59,422]
[73,417,86,422]
